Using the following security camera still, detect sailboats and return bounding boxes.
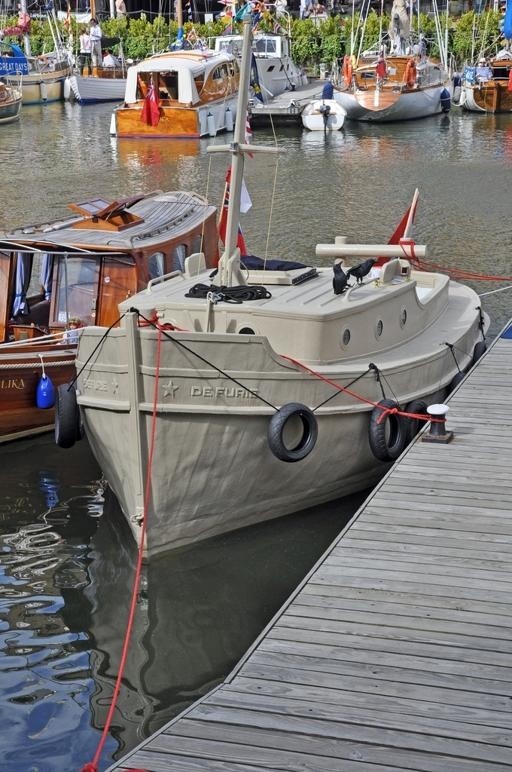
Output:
[2,2,74,106]
[313,2,459,120]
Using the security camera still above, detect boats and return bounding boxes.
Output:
[214,4,331,117]
[302,99,347,131]
[75,15,489,558]
[0,192,217,448]
[2,70,24,120]
[448,2,511,115]
[65,42,141,105]
[110,49,239,139]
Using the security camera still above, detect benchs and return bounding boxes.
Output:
[375,50,389,80]
[139,77,165,129]
[372,188,419,267]
[218,105,255,257]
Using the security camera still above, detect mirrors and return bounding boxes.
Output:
[333,261,355,295]
[347,258,379,285]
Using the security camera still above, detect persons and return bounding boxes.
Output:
[101,47,122,72]
[115,0,128,20]
[222,0,512,33]
[78,25,95,78]
[102,48,109,62]
[88,16,103,68]
[476,57,494,84]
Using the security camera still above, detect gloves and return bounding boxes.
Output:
[0,192,217,448]
[110,49,239,139]
[302,99,347,131]
[75,15,489,558]
